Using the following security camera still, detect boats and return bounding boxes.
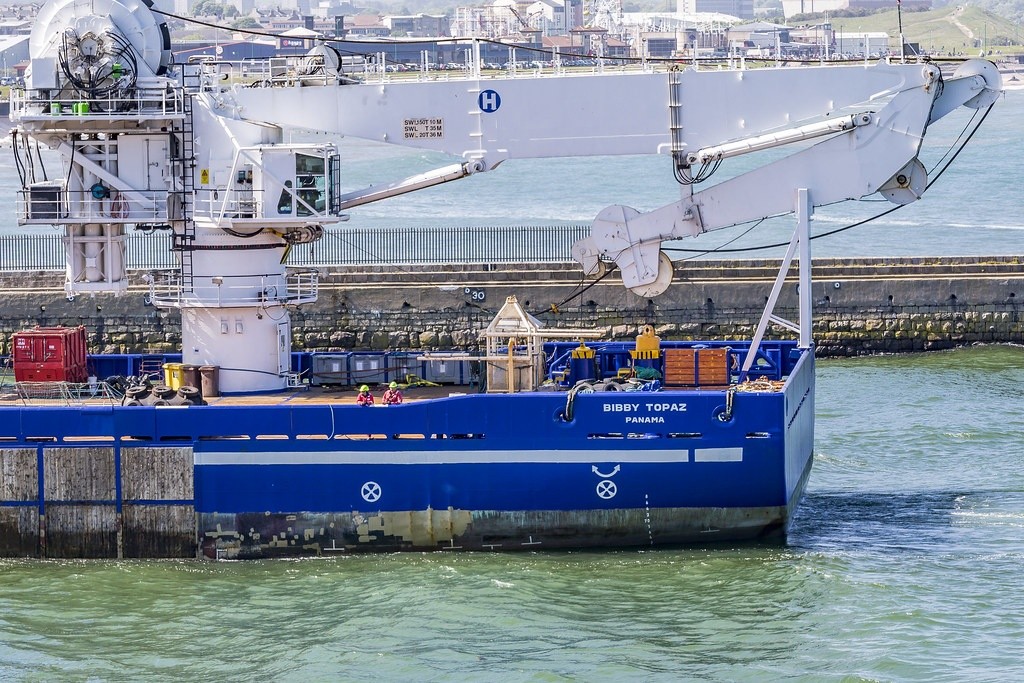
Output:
[0,0,1005,559]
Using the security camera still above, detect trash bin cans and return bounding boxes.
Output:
[162,363,220,398]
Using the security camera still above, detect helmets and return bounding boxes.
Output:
[359,385,369,391]
[389,381,398,387]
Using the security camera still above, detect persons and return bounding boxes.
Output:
[357,385,374,440]
[381,380,402,438]
